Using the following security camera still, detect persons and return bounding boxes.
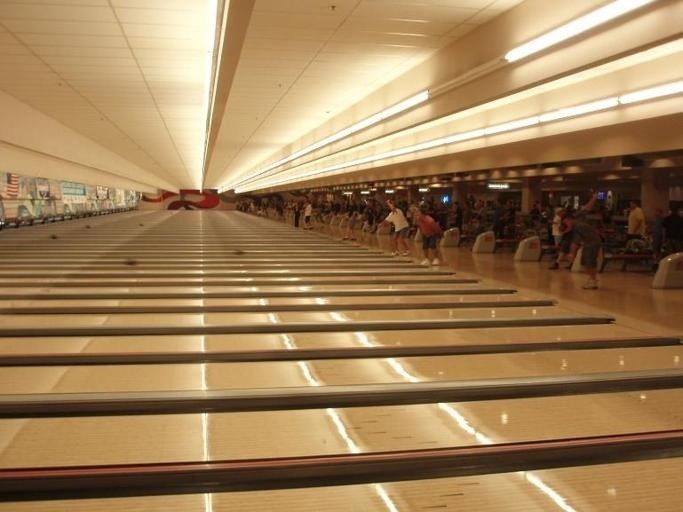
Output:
[452,190,683,270]
[235,189,449,266]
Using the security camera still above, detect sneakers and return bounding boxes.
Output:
[582,278,599,289]
[392,251,400,256]
[565,263,572,269]
[432,258,441,265]
[548,262,559,269]
[402,250,410,256]
[420,259,432,265]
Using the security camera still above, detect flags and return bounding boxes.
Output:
[6,173,20,199]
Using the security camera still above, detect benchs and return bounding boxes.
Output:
[603,251,653,271]
